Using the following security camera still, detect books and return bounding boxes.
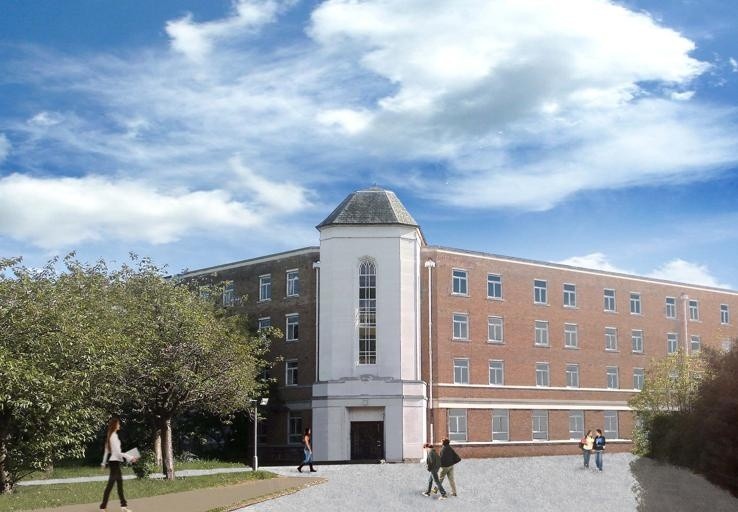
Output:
[125,447,141,462]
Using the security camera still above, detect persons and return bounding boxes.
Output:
[422,443,447,501]
[297,428,317,472]
[581,430,594,470]
[431,439,461,496]
[100,418,137,512]
[593,429,606,473]
